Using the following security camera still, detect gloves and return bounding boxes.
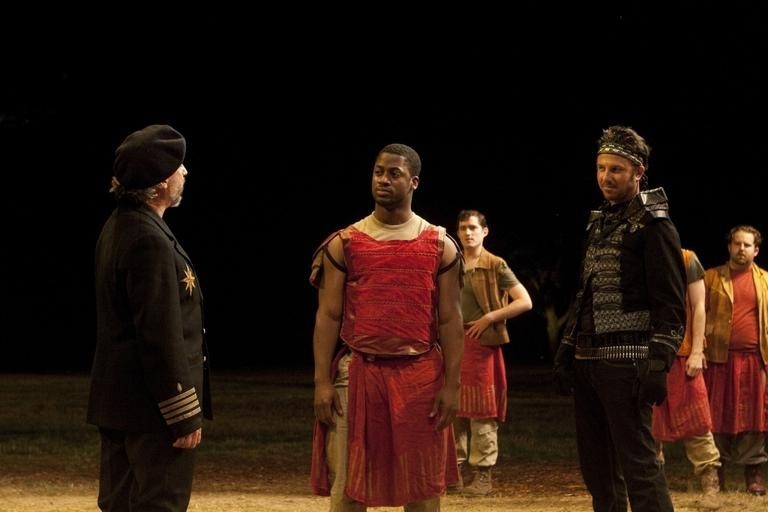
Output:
[632,342,675,406]
[555,343,576,377]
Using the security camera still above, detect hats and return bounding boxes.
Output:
[113,125,186,190]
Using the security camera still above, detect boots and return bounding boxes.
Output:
[700,466,719,509]
[446,464,464,495]
[744,464,766,496]
[461,466,492,496]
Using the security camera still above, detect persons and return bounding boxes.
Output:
[549,124,693,512]
[83,120,213,512]
[451,208,535,495]
[646,245,722,497]
[701,223,768,499]
[305,143,464,511]
